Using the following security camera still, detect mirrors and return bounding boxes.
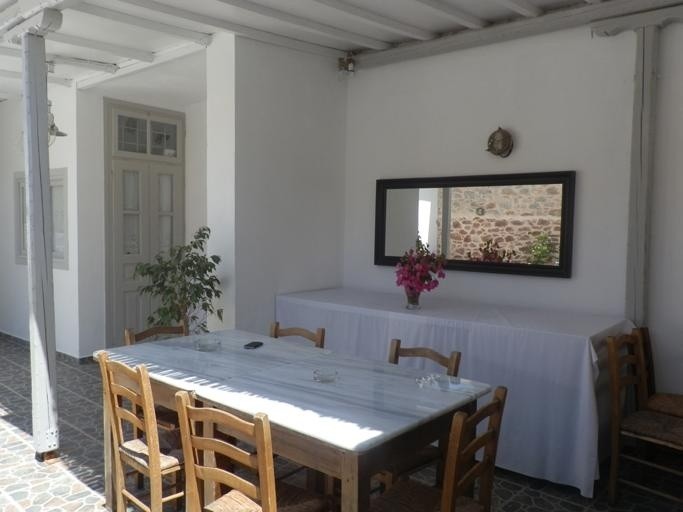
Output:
[374,171,577,278]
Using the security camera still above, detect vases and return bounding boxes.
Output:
[404,287,422,310]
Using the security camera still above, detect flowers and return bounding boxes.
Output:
[394,230,448,293]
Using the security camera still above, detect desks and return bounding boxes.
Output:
[93,328,492,512]
[274,287,637,499]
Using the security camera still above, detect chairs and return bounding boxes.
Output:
[125,315,180,489]
[366,385,507,512]
[635,327,683,418]
[607,328,683,507]
[388,339,461,376]
[270,322,325,348]
[174,390,335,512]
[98,350,185,512]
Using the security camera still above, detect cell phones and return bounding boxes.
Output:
[244,341,264,349]
